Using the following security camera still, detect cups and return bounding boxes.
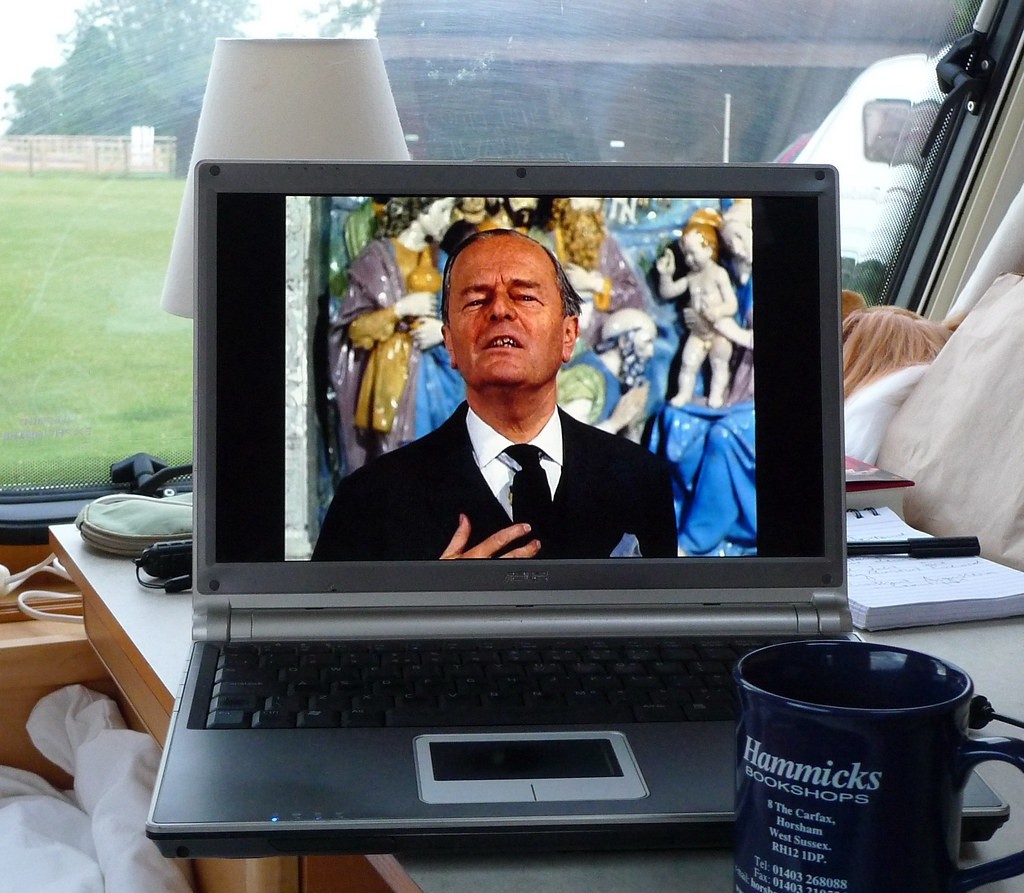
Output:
[730,641,1024,893]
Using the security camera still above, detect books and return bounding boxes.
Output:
[845,455,914,523]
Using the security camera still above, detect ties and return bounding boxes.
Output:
[499,443,554,525]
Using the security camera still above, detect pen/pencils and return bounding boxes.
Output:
[845,535,981,560]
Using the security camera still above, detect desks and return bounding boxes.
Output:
[49,521,1024,891]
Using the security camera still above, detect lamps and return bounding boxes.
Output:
[160,34,414,321]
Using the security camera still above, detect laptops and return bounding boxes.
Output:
[145,157,1010,860]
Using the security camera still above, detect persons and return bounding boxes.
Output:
[323,193,466,478]
[654,221,737,413]
[532,202,648,362]
[659,203,751,547]
[557,304,659,446]
[474,198,552,252]
[303,229,681,559]
